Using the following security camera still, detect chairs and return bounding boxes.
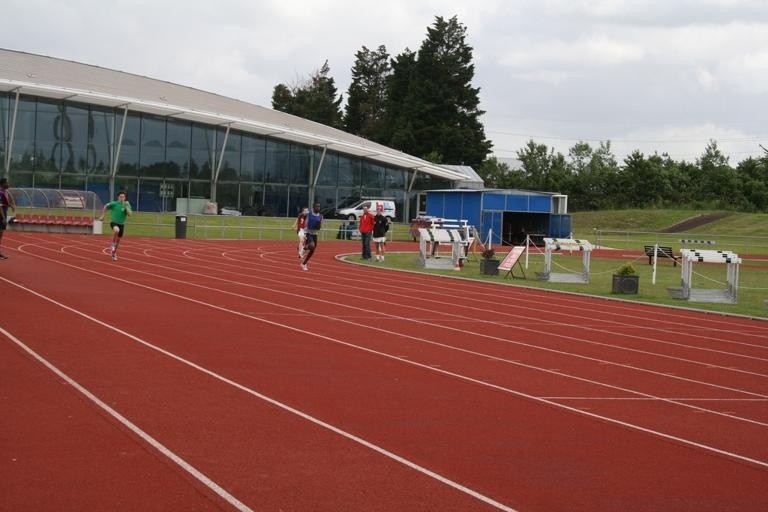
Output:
[13,213,94,233]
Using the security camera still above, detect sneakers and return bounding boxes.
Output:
[300,263,308,271]
[112,256,117,261]
[300,246,306,259]
[111,242,116,252]
[0,254,8,260]
[376,255,385,262]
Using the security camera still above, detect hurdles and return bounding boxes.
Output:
[535,237,594,285]
[665,249,742,305]
[411,215,477,271]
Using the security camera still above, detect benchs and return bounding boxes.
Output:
[643,245,683,267]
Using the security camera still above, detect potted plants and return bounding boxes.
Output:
[479,248,501,276]
[610,263,639,295]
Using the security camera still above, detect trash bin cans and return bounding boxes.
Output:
[175,215,187,240]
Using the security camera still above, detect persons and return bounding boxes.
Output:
[359,207,375,260]
[297,202,322,270]
[346,221,354,240]
[336,222,345,240]
[1,178,15,259]
[294,208,308,258]
[99,192,133,261]
[372,207,389,263]
